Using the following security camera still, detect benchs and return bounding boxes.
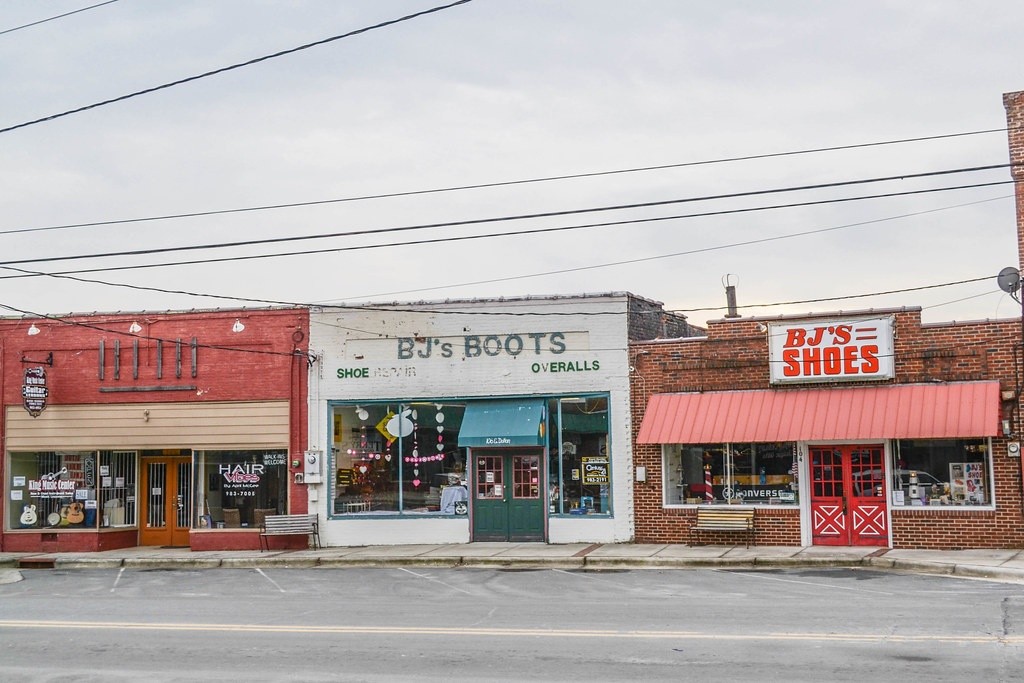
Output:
[688,507,757,548]
[259,513,322,553]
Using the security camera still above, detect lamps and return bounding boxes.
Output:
[130,317,147,333]
[28,321,58,335]
[889,315,900,340]
[757,322,768,337]
[143,410,150,423]
[232,315,250,332]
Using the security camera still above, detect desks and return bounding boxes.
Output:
[343,501,369,513]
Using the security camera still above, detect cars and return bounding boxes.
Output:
[853,468,950,507]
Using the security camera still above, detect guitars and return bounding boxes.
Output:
[20,490,38,525]
[59,480,84,526]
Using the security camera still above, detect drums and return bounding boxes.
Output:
[48,512,61,526]
